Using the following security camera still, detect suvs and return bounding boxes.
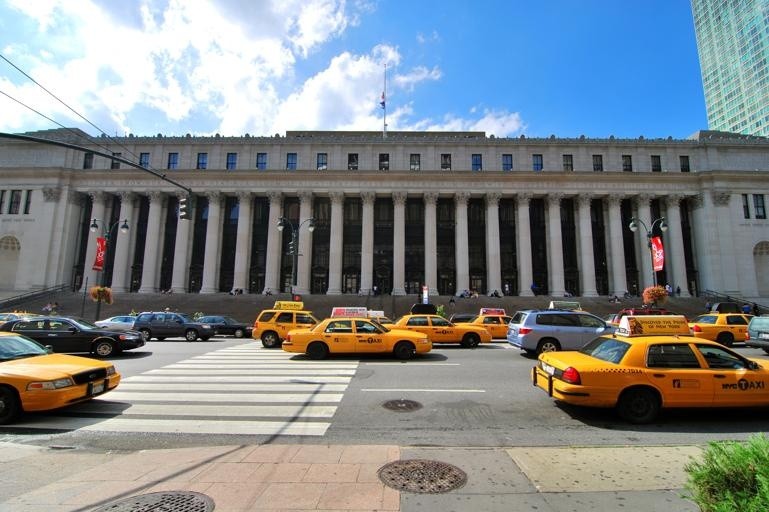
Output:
[742,313,768,353]
[249,300,321,349]
[507,308,618,359]
[132,308,213,342]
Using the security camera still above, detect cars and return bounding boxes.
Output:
[367,311,393,329]
[1,312,62,327]
[0,316,148,357]
[281,306,434,359]
[593,305,695,332]
[531,313,769,422]
[447,311,476,322]
[194,315,254,338]
[466,307,514,340]
[551,300,620,328]
[687,301,757,349]
[0,331,121,425]
[92,315,136,331]
[379,304,493,347]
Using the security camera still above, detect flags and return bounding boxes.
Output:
[381,90,385,110]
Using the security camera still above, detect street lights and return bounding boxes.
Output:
[86,214,130,322]
[276,213,317,294]
[626,214,673,308]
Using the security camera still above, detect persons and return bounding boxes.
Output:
[608,290,630,304]
[43,302,58,312]
[449,284,509,307]
[706,296,759,316]
[665,283,680,296]
[266,288,271,296]
[232,288,240,296]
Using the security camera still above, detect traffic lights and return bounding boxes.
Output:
[178,196,189,220]
[293,293,302,301]
